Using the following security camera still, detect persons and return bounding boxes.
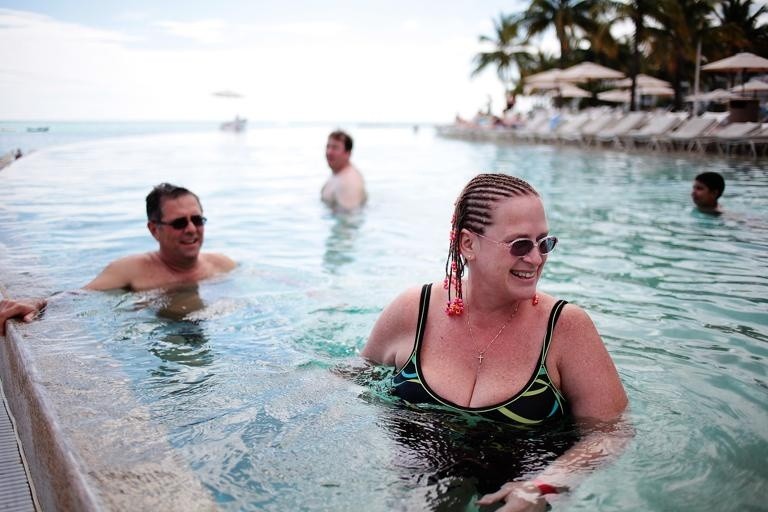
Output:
[334,172,636,510]
[687,171,732,217]
[319,131,368,214]
[1,182,341,341]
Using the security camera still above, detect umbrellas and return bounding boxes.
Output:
[522,52,768,121]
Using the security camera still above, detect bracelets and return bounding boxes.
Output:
[530,478,561,502]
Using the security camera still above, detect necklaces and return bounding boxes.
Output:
[464,277,520,365]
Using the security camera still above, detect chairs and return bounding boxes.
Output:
[520,105,768,163]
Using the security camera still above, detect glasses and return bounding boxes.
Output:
[468,228,558,259]
[153,214,207,230]
